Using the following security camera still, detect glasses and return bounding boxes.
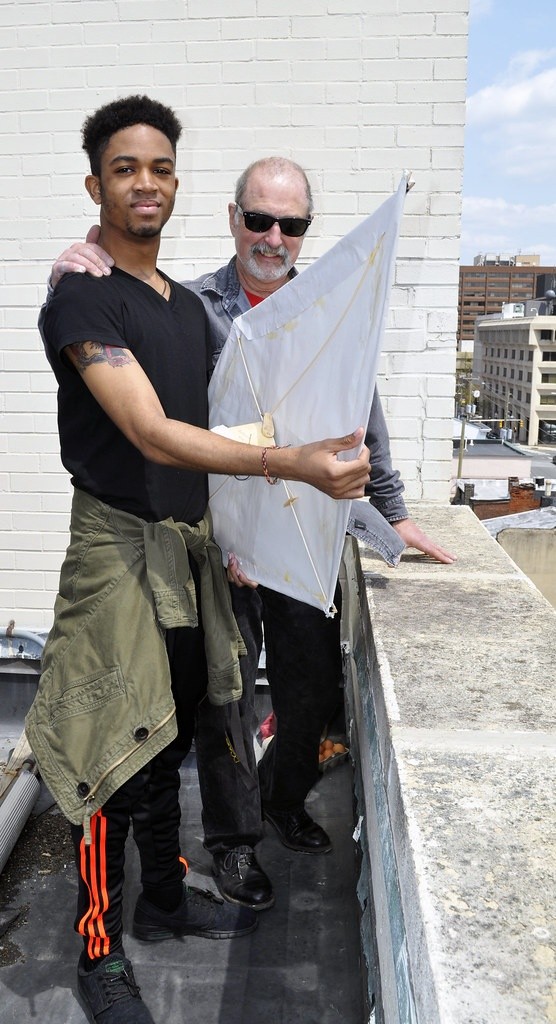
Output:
[234,201,314,237]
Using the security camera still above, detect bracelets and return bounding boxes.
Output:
[261,445,282,485]
[46,273,56,300]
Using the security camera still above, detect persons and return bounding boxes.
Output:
[24,94,372,1024]
[47,158,460,909]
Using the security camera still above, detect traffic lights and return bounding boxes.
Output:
[520,420,523,428]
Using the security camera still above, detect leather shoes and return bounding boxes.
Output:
[260,801,333,856]
[211,845,275,911]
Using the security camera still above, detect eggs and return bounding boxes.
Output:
[318,739,345,762]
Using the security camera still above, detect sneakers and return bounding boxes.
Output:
[133,886,260,941]
[78,947,155,1024]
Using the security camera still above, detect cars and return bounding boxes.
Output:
[545,424,556,437]
[0,626,50,660]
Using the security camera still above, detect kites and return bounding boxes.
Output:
[207,170,417,621]
